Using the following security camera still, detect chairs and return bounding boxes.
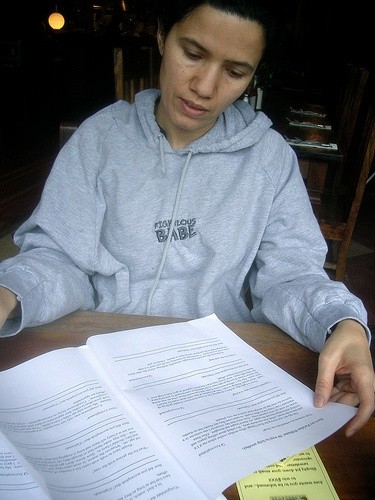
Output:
[312,64,375,281]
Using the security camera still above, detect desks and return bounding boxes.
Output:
[0,310,375,500]
[268,100,342,218]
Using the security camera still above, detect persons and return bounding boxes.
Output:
[0,0,375,439]
